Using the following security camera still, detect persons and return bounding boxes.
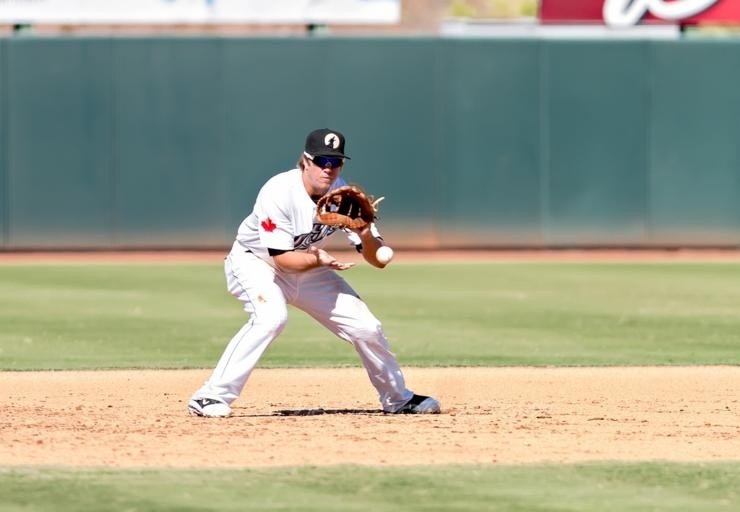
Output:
[186,126,444,418]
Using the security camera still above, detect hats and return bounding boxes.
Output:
[304,128,351,159]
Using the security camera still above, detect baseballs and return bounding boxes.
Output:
[376,246,393,264]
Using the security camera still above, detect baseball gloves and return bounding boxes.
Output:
[315,186,386,229]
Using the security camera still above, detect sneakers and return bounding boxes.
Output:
[188,399,231,418]
[395,394,440,414]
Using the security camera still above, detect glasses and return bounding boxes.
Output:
[304,149,343,168]
